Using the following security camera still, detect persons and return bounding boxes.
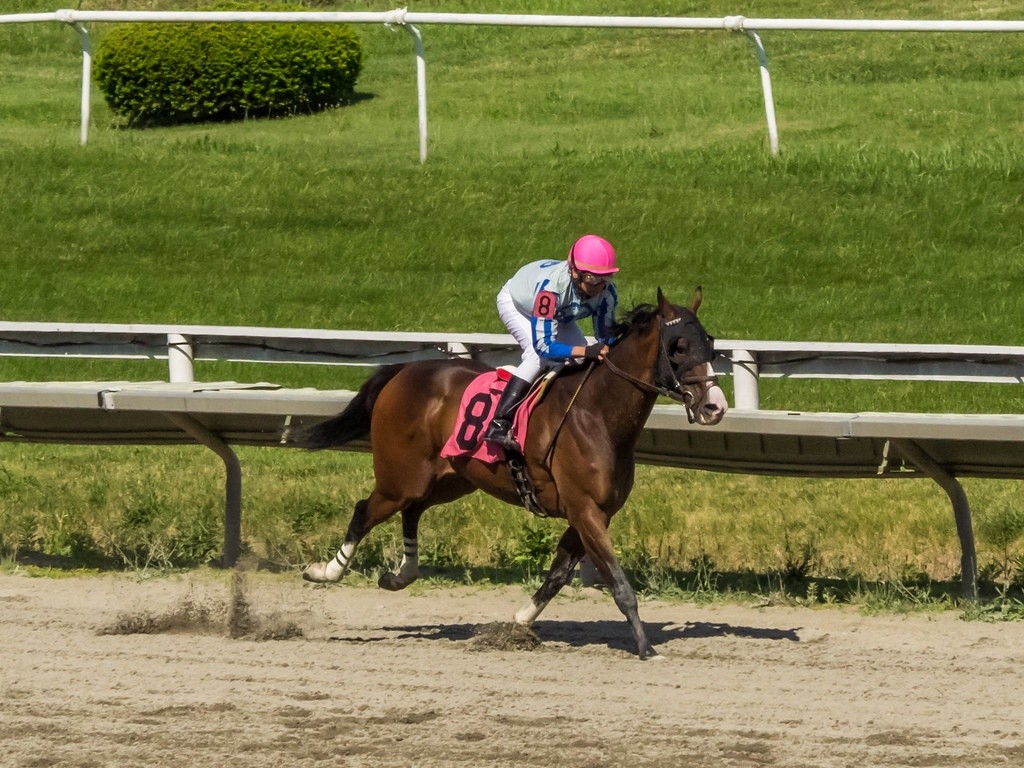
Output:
[482,234,620,453]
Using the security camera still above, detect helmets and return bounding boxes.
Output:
[567,235,620,276]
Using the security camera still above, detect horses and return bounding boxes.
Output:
[289,283,730,662]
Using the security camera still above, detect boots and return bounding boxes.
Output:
[482,375,535,451]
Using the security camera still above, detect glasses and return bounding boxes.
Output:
[582,273,612,290]
